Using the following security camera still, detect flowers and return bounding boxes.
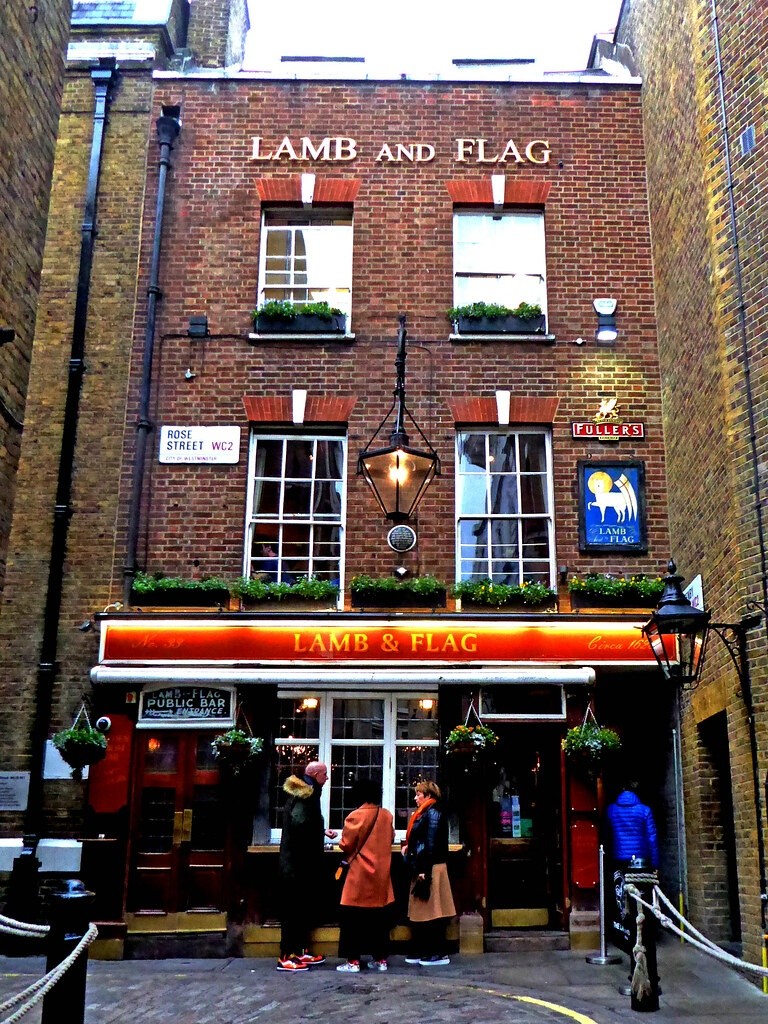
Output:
[561,720,622,759]
[444,725,500,755]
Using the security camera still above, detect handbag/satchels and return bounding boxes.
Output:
[335,860,350,885]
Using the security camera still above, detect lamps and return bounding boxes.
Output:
[594,317,619,341]
[357,316,441,521]
[188,316,208,337]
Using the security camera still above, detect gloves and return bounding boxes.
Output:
[411,873,432,904]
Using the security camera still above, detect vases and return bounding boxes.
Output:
[571,746,609,760]
[453,742,477,752]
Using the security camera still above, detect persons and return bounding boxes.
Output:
[608,781,659,874]
[277,761,339,971]
[401,782,456,965]
[336,781,396,972]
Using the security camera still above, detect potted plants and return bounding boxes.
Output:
[448,302,546,333]
[251,300,347,334]
[52,697,107,767]
[210,730,264,775]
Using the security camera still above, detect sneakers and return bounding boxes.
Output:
[336,961,360,972]
[298,953,325,964]
[419,954,451,965]
[367,959,388,971]
[276,955,308,970]
[405,953,431,964]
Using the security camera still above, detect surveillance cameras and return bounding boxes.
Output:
[96,716,111,731]
